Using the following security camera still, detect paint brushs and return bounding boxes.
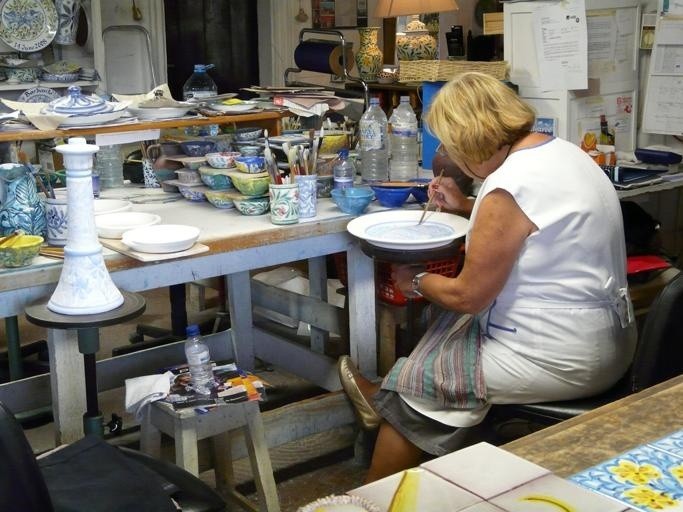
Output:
[418,169,444,225]
[264,126,324,184]
[370,182,429,188]
[17,149,56,198]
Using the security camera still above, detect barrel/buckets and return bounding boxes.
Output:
[182,64,220,137]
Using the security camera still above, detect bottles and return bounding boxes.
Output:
[52,1,80,47]
[334,96,418,208]
[184,324,216,395]
[183,64,218,101]
[2,162,46,237]
[599,121,615,145]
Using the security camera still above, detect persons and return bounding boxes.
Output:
[365,72,635,486]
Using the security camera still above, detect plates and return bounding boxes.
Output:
[347,211,472,251]
[93,211,163,241]
[5,66,40,85]
[127,103,198,121]
[42,59,82,74]
[122,223,200,254]
[41,71,82,82]
[0,0,59,53]
[15,107,127,130]
[94,197,134,217]
[16,88,63,105]
[208,98,279,113]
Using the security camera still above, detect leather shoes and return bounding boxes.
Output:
[337,355,381,431]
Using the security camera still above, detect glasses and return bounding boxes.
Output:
[435,143,448,158]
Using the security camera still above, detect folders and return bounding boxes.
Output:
[598,165,669,183]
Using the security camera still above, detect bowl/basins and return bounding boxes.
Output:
[174,126,347,225]
[0,234,45,269]
[331,178,432,214]
[377,58,378,85]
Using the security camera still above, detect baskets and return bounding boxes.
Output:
[335,253,459,306]
[398,60,509,84]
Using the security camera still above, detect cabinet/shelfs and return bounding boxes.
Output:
[505,0,641,150]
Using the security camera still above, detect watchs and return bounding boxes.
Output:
[411,272,428,296]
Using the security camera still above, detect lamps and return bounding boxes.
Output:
[373,0,459,60]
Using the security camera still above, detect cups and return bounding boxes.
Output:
[44,197,69,248]
